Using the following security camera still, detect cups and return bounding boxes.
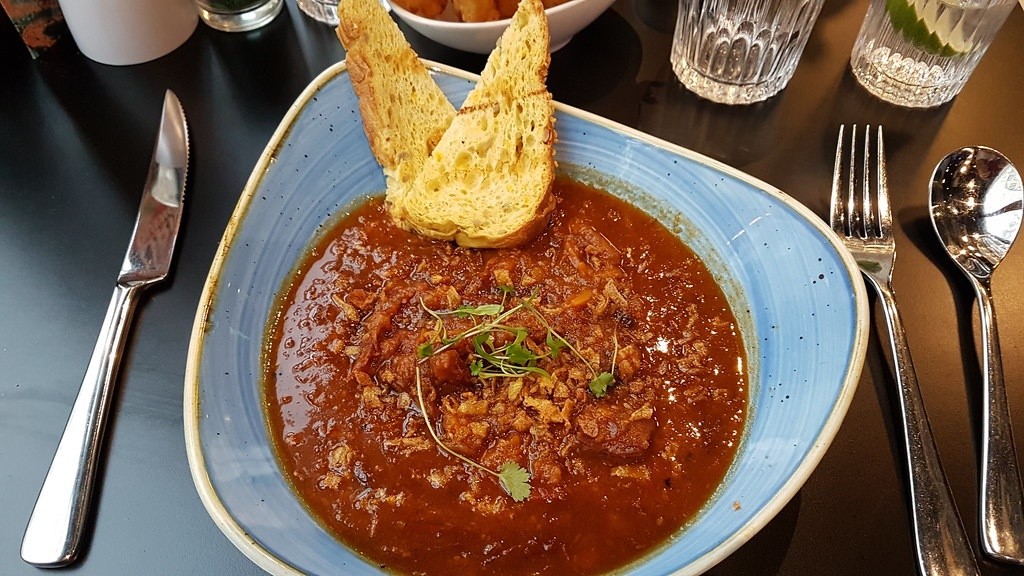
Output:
[296,0,391,25]
[851,0,1019,109]
[197,0,285,33]
[670,0,826,104]
[56,1,200,67]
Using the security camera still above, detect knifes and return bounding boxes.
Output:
[21,87,191,566]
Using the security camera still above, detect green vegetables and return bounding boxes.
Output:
[414,285,617,501]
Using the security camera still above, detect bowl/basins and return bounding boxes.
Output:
[386,0,615,54]
[182,53,871,576]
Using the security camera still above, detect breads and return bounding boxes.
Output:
[335,0,558,249]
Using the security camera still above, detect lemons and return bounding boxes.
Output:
[888,0,973,56]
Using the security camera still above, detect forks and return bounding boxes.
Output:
[830,123,983,576]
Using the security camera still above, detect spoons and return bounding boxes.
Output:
[928,145,1024,570]
